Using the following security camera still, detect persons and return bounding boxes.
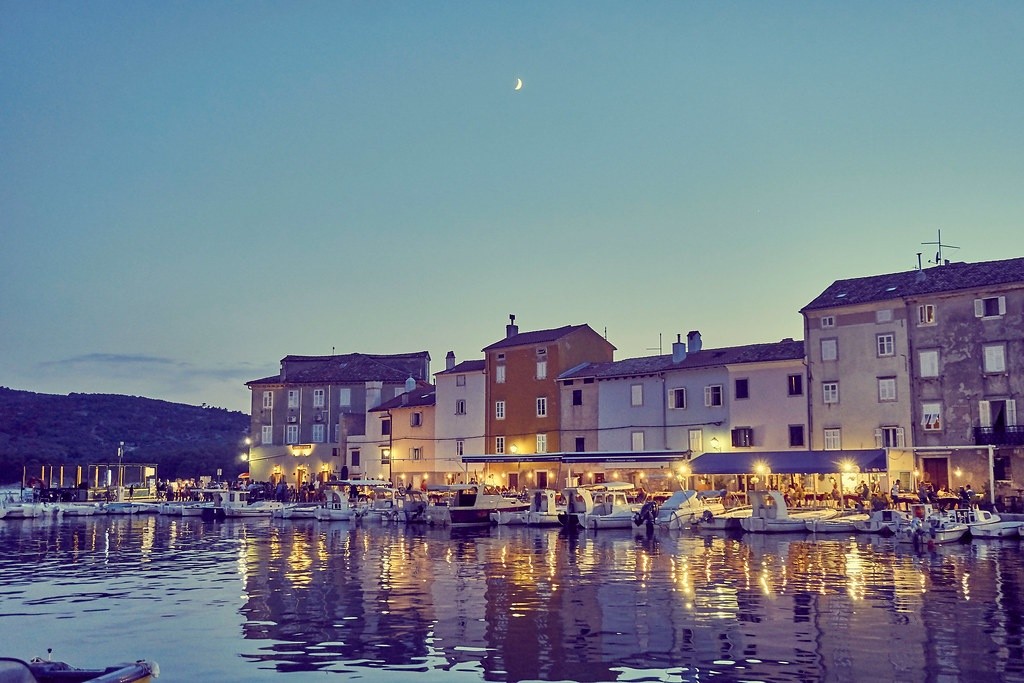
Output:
[456,478,478,496]
[870,494,890,518]
[766,485,778,501]
[157,477,327,502]
[919,481,950,514]
[854,481,869,509]
[419,479,427,492]
[784,483,803,507]
[631,488,646,503]
[892,479,904,511]
[487,485,528,502]
[747,482,753,504]
[958,484,975,515]
[832,483,851,508]
[871,476,880,494]
[94,489,117,504]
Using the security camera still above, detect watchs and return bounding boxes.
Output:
[128,483,136,501]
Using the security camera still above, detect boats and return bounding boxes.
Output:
[940,511,1001,532]
[557,481,637,529]
[0,490,363,520]
[354,487,430,522]
[423,485,531,525]
[892,521,968,545]
[631,490,725,529]
[853,509,913,534]
[806,512,870,533]
[969,521,1023,536]
[489,489,564,525]
[739,490,838,533]
[699,508,753,530]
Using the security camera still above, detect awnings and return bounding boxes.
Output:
[684,450,888,475]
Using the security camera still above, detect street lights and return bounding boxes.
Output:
[118,441,124,486]
